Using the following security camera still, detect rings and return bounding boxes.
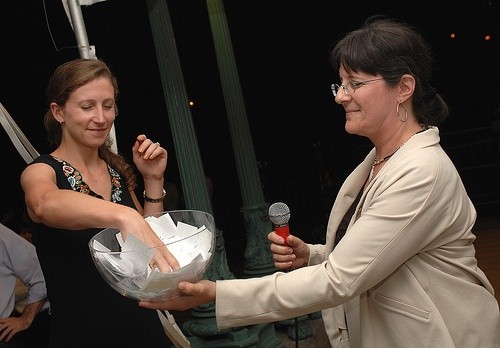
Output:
[155,142,160,146]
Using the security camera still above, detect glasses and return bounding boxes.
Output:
[331,74,403,96]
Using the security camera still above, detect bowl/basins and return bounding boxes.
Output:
[89,209,216,303]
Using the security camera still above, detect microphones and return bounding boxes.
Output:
[269,202,293,272]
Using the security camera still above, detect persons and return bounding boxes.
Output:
[139,21,500,348]
[20,59,167,348]
[0,223,50,348]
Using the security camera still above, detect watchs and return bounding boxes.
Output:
[143,189,166,203]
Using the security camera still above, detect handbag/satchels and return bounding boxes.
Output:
[157,309,191,348]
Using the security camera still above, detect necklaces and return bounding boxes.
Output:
[369,127,425,182]
